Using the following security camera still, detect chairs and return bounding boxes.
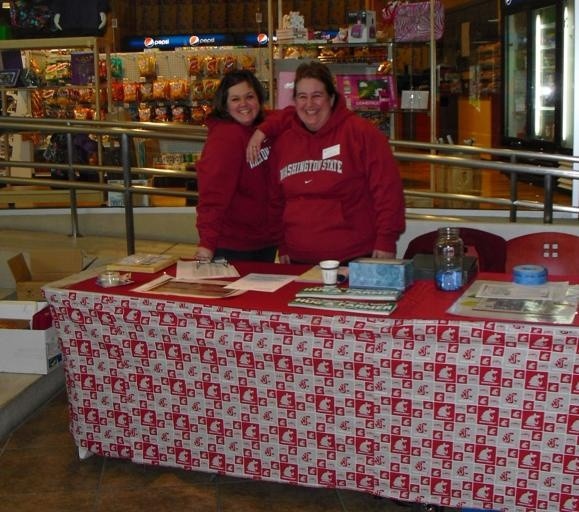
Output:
[403,224,578,278]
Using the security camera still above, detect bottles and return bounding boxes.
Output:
[434,227,464,291]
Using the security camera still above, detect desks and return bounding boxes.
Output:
[38,259,579,512]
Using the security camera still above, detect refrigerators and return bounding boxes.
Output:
[501,0,575,188]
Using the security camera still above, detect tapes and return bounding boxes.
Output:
[98,272,120,288]
[512,265,547,286]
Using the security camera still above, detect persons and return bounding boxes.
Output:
[264,60,407,265]
[193,69,296,265]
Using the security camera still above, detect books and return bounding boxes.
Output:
[107,251,178,274]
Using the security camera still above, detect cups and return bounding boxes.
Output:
[318,260,340,287]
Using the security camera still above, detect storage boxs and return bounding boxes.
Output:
[1,244,89,376]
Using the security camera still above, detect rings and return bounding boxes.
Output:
[252,146,257,149]
